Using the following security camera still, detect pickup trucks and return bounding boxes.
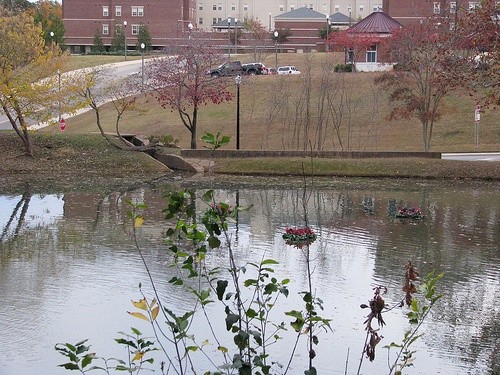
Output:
[207,60,260,78]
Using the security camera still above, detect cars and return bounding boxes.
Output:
[277,65,300,75]
[241,63,277,74]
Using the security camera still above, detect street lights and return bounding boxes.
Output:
[234,73,242,150]
[140,43,146,90]
[274,30,279,71]
[187,23,194,79]
[233,18,238,54]
[227,17,231,59]
[325,14,331,51]
[48,31,55,58]
[56,69,62,122]
[123,20,128,61]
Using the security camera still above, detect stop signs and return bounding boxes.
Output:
[59,119,67,130]
